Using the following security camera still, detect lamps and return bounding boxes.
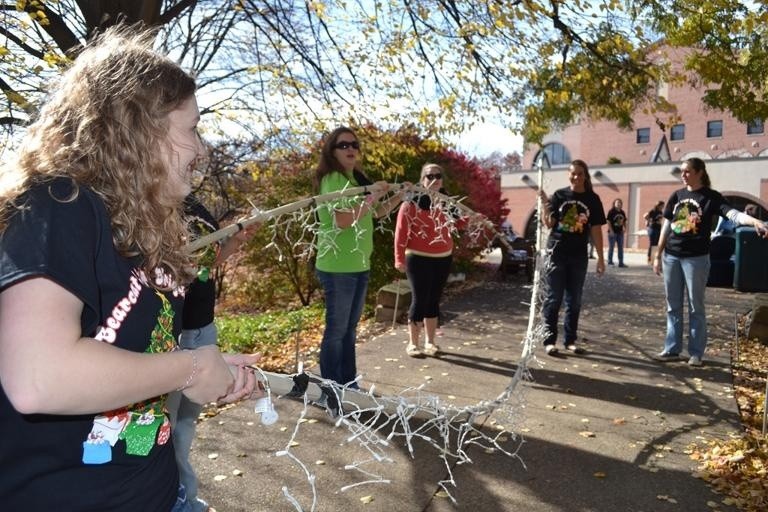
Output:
[640,150,650,158]
[751,140,768,148]
[711,143,730,155]
[671,167,682,175]
[674,146,688,156]
[522,175,529,180]
[593,171,602,177]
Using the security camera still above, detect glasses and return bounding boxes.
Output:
[425,173,442,180]
[330,140,359,151]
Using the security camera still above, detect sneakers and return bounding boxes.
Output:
[545,343,560,355]
[687,354,703,367]
[404,343,425,358]
[653,349,680,362]
[618,263,629,268]
[563,342,585,355]
[608,262,615,268]
[422,342,444,358]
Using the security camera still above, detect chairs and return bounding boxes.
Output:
[494,237,533,284]
[706,234,737,288]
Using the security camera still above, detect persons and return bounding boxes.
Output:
[588,242,597,260]
[540,160,606,355]
[394,164,486,356]
[160,158,262,498]
[607,199,629,267]
[314,127,412,409]
[653,156,768,366]
[0,31,269,511]
[643,201,664,262]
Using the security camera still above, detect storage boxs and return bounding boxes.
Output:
[735,230,768,294]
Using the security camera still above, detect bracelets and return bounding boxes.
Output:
[176,351,196,392]
[655,255,662,260]
